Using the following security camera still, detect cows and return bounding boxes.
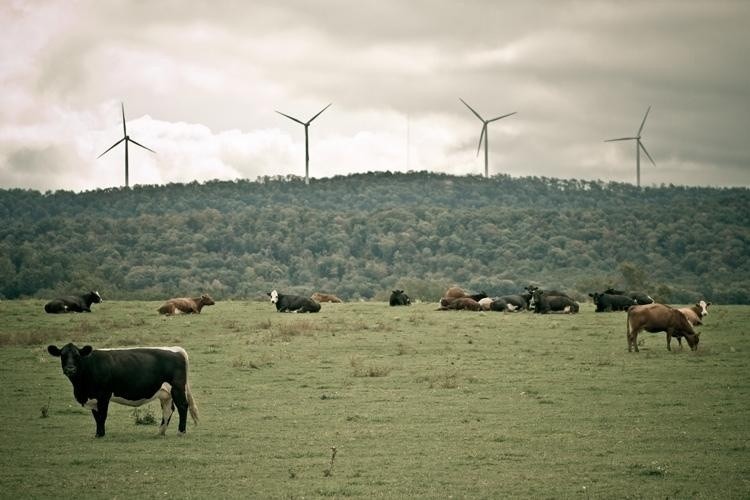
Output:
[44,290,103,315]
[47,341,200,438]
[434,286,579,315]
[310,292,343,304]
[677,300,712,328]
[389,289,411,306]
[266,289,321,314]
[588,287,656,313]
[156,293,215,317]
[626,303,701,353]
